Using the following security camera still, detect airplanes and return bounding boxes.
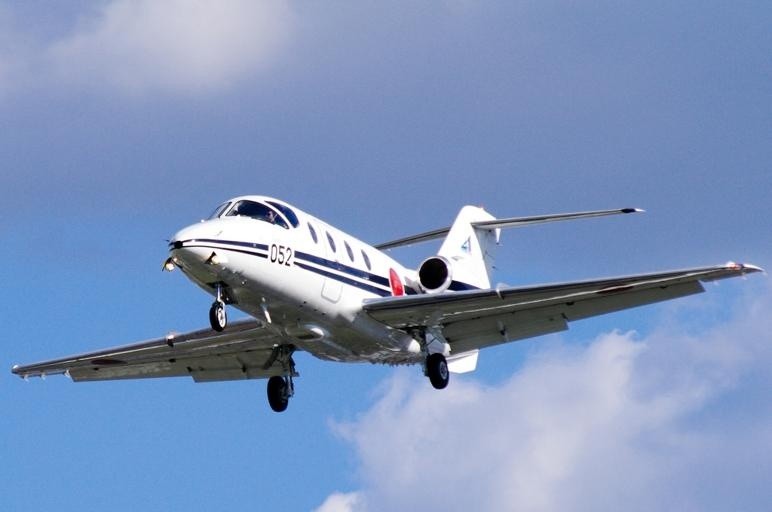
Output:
[10,193,764,411]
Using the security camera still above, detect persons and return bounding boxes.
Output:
[262,210,278,224]
